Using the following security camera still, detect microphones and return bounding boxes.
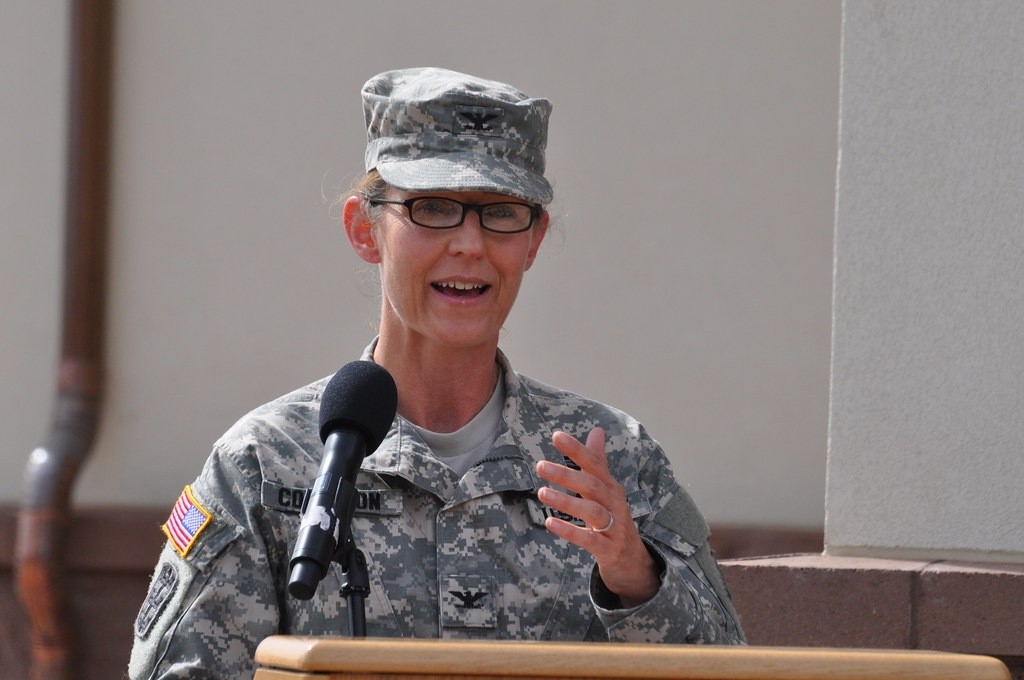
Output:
[289,360,399,601]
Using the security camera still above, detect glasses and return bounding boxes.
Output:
[364,196,542,233]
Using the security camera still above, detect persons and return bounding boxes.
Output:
[128,67,756,680]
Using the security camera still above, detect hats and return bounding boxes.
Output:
[361,66,554,205]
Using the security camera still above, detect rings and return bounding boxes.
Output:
[593,510,614,533]
